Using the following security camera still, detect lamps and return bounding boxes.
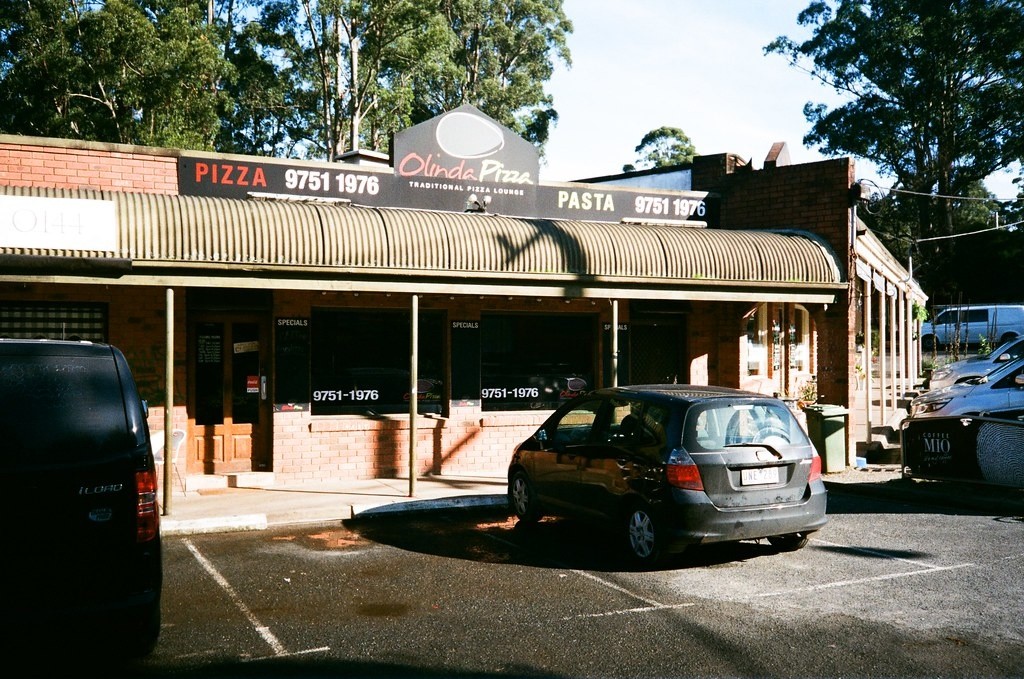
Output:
[464,192,492,213]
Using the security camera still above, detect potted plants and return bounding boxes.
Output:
[852,365,866,390]
[797,383,817,411]
[923,356,939,378]
[854,332,865,352]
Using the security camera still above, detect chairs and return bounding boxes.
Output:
[610,416,651,448]
[150,428,187,496]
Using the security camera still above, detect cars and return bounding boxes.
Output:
[504,384,829,569]
[909,354,1024,420]
[928,335,1024,393]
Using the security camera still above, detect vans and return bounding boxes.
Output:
[920,305,1024,355]
[0,341,166,665]
